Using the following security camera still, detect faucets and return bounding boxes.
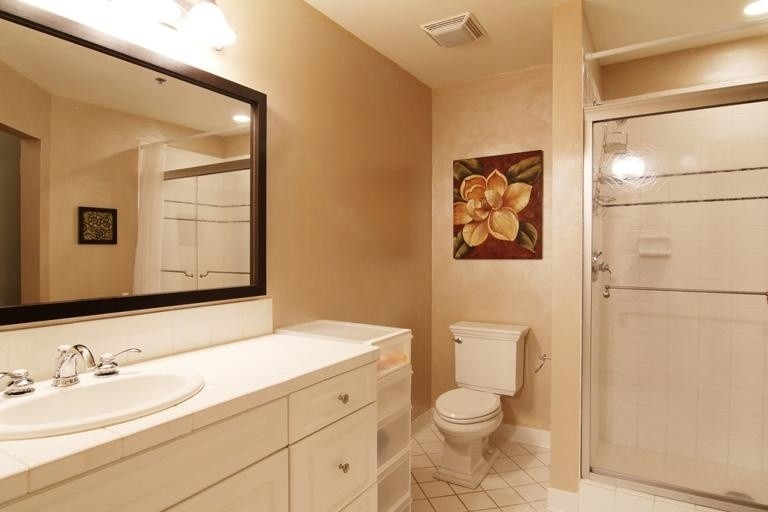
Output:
[50,341,98,388]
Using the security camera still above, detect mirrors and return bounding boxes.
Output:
[0,0,268,324]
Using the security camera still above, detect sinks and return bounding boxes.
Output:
[0,365,206,445]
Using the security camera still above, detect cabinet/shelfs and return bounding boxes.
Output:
[2,360,380,510]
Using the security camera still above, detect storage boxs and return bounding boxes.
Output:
[275,319,413,511]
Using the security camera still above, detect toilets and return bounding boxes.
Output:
[430,316,531,493]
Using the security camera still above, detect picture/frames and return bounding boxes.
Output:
[76,207,119,244]
